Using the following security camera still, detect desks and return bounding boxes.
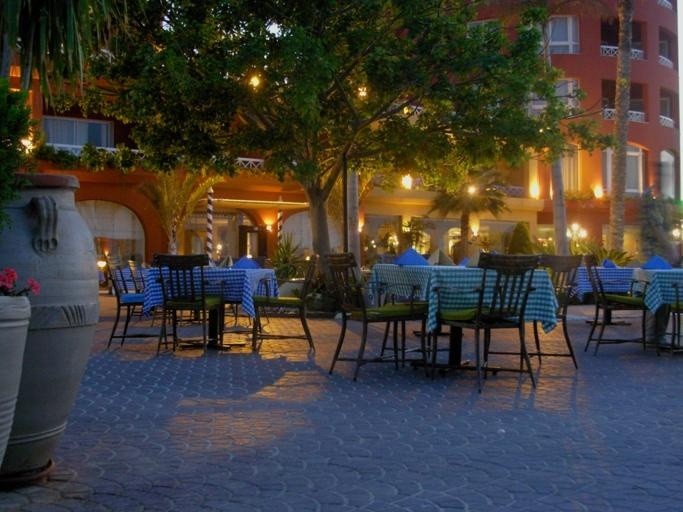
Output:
[576,266,682,346]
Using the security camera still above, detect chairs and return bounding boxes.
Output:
[584,255,648,357]
[325,253,582,389]
[251,254,317,352]
[106,252,256,356]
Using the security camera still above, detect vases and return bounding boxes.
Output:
[0,294,32,467]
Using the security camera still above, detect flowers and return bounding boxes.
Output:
[0,265,41,299]
